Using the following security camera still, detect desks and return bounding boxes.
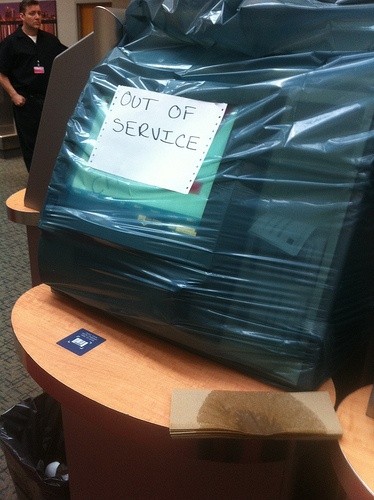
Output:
[327,383,374,500]
[6,188,43,288]
[11,281,337,500]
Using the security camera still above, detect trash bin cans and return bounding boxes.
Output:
[0,392,70,500]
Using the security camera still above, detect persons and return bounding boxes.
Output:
[0,0,69,175]
